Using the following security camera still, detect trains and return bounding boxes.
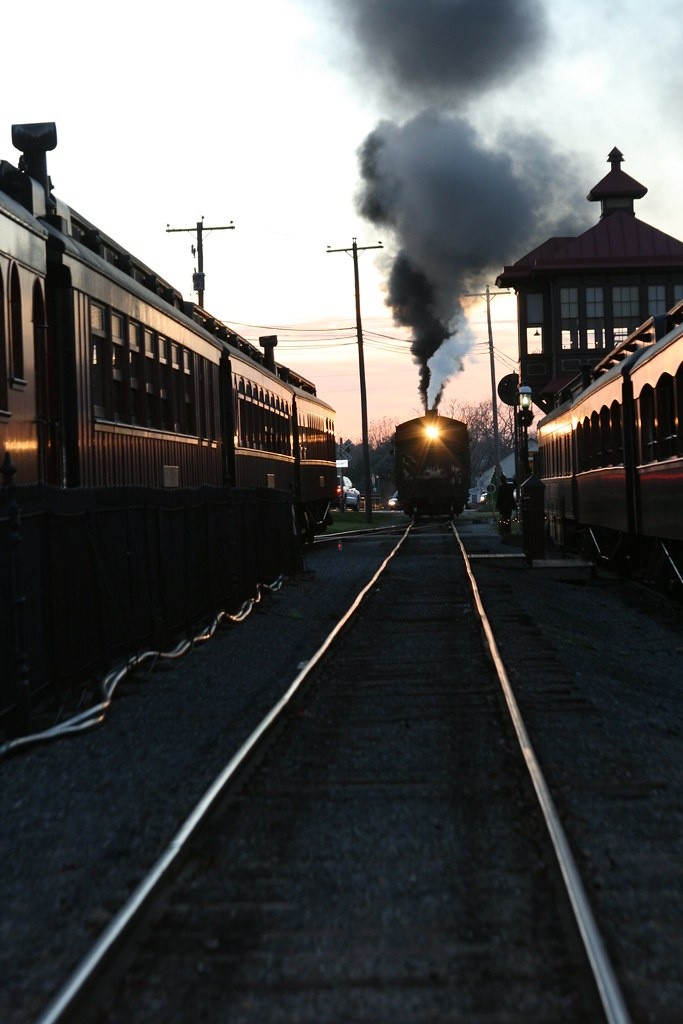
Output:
[387,409,472,520]
[534,299,683,592]
[0,121,338,742]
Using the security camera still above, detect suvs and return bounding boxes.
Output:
[334,476,361,513]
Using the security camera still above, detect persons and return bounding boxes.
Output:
[496,476,517,533]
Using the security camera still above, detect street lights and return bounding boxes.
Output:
[516,383,533,478]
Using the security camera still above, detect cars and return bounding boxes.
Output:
[387,490,401,510]
